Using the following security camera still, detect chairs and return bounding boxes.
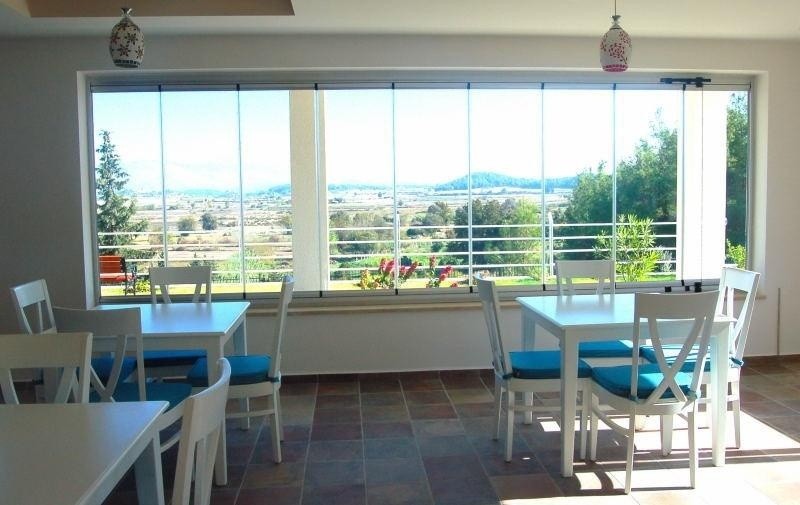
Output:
[634,266,760,456]
[556,260,642,365]
[50,304,191,453]
[472,276,591,463]
[171,358,232,505]
[124,268,211,388]
[10,279,136,404]
[99,255,136,296]
[591,291,720,494]
[187,277,293,463]
[0,332,93,404]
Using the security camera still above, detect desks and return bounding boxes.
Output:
[516,292,739,478]
[0,401,171,505]
[39,302,251,486]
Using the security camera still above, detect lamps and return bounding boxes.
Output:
[600,0,633,72]
[108,0,144,68]
